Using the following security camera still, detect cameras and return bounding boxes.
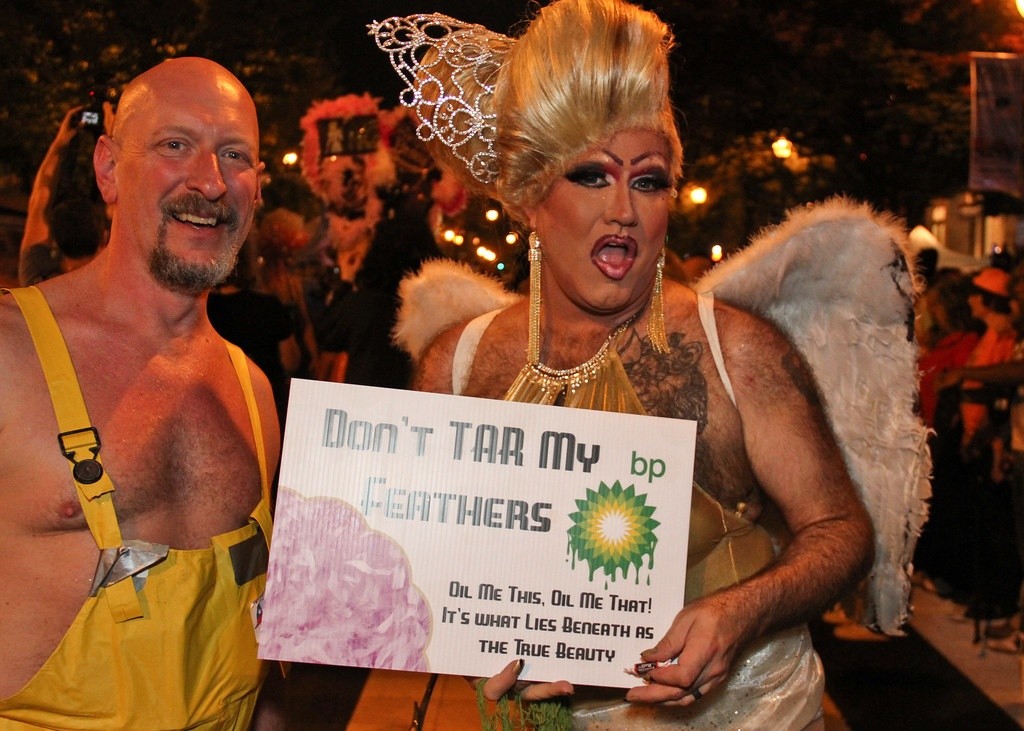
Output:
[78,110,105,128]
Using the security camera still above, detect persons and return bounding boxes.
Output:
[663,238,751,287]
[365,0,875,731]
[19,64,445,515]
[822,245,1024,654]
[0,56,283,731]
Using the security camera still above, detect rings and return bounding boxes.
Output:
[693,690,703,701]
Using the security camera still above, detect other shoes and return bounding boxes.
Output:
[823,603,857,623]
[834,625,888,642]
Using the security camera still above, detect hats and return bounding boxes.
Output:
[264,208,309,249]
[973,268,1011,296]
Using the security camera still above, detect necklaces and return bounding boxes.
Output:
[501,314,649,416]
